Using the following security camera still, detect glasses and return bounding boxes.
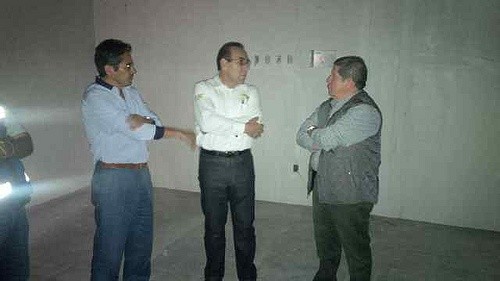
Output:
[228,58,250,65]
[114,63,134,70]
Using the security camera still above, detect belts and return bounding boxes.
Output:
[201,149,249,157]
[100,163,145,169]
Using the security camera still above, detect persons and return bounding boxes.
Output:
[0,99,35,281]
[191,42,265,281]
[81,38,197,280]
[296,56,383,281]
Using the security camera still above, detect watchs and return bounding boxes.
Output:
[307,125,318,134]
[143,116,155,123]
